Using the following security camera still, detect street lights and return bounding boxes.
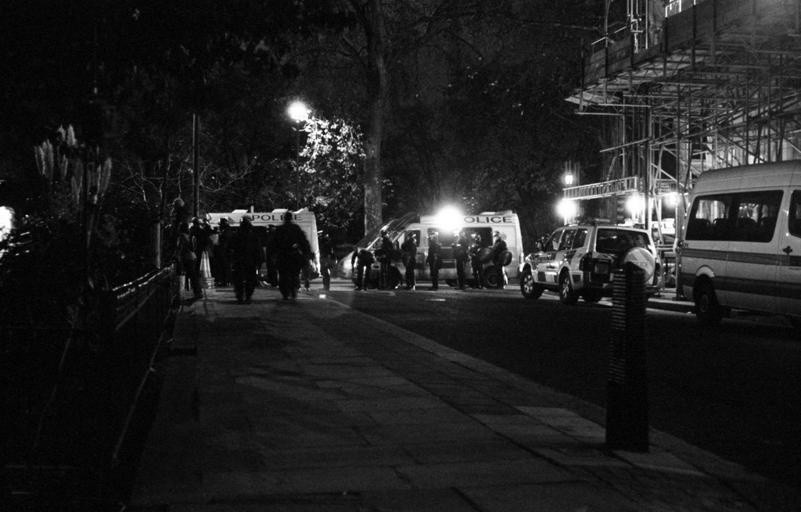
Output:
[286,97,309,215]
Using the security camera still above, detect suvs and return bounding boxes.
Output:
[510,217,663,309]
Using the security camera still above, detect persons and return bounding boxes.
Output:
[266,212,317,303]
[426,232,442,291]
[175,217,271,304]
[614,215,634,253]
[624,246,655,318]
[401,232,417,291]
[469,234,487,290]
[489,230,507,289]
[536,234,553,252]
[452,232,468,289]
[652,224,665,296]
[319,230,334,290]
[351,247,373,291]
[633,223,647,250]
[374,229,393,291]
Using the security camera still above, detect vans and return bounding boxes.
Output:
[672,153,801,323]
[336,206,529,285]
[184,208,327,291]
